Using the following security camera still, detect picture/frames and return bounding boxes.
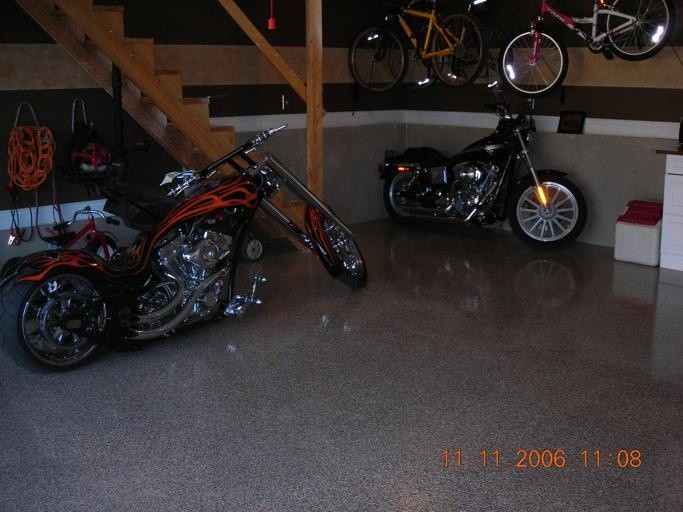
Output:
[557,110,584,134]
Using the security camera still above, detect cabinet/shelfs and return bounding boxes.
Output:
[660,155,683,271]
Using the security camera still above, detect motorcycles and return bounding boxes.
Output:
[1,114,370,377]
[374,84,590,251]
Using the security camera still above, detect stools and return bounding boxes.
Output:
[614,200,662,266]
